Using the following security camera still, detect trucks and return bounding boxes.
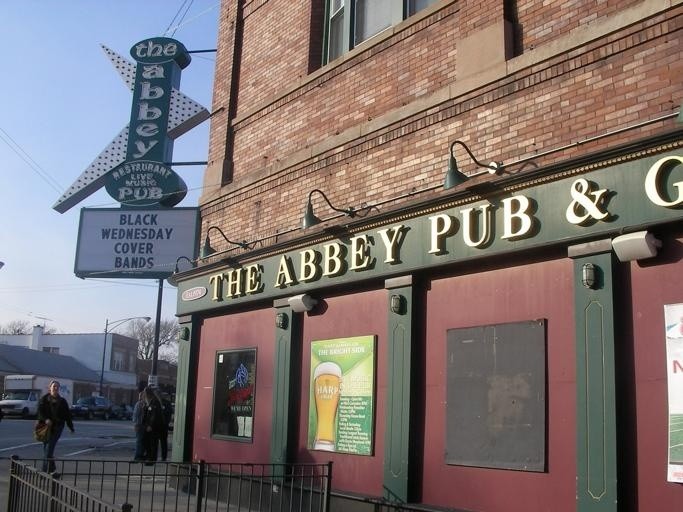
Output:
[0,374,74,419]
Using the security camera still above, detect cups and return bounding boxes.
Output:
[313,361,342,452]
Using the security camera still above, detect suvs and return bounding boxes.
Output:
[70,395,114,420]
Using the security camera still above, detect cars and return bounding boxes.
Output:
[112,403,133,420]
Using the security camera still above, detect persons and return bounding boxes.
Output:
[128,383,171,466]
[36,380,74,473]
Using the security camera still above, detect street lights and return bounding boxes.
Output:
[99,314,150,398]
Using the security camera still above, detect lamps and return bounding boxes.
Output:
[275,312,287,329]
[287,294,320,313]
[612,231,664,262]
[443,141,499,191]
[300,189,354,230]
[178,327,188,340]
[391,294,401,312]
[199,226,248,259]
[167,257,196,287]
[582,262,595,288]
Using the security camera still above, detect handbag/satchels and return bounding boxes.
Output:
[35,424,51,443]
